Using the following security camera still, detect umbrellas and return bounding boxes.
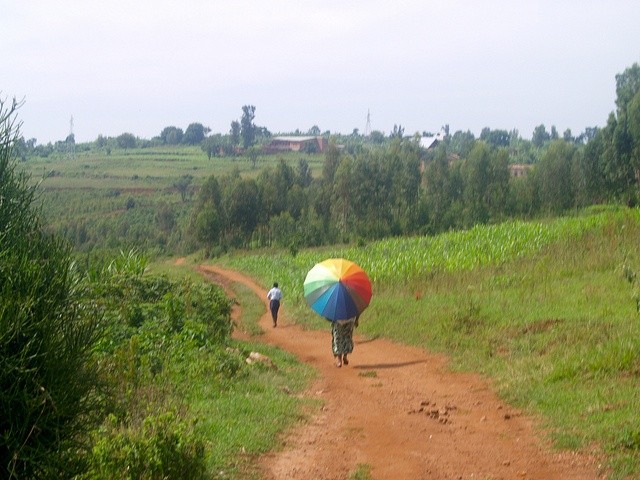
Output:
[303,258,373,320]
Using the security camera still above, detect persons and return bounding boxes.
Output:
[325,315,359,368]
[267,283,284,327]
